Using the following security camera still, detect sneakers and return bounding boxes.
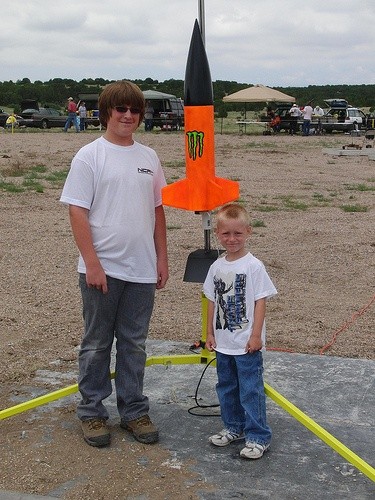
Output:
[80,418,111,446]
[208,428,246,447]
[120,414,159,444]
[240,440,272,459]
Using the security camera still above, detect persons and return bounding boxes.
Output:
[62,97,80,133]
[61,80,169,445]
[203,204,278,459]
[289,104,301,134]
[301,102,313,136]
[314,106,324,115]
[79,102,87,131]
[144,103,154,130]
[269,112,281,135]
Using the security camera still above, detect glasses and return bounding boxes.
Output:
[114,106,141,114]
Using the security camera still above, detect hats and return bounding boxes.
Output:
[292,104,297,107]
[67,97,73,100]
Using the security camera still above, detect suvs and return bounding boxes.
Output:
[142,98,185,132]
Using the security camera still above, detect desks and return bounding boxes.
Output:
[82,116,102,132]
[235,120,272,136]
[149,117,180,131]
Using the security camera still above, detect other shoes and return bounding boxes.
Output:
[61,128,67,132]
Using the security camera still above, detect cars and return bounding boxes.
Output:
[317,98,369,134]
[258,107,301,121]
[18,98,74,130]
[0,109,23,127]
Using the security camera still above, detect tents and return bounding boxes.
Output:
[221,85,296,135]
[143,90,180,131]
